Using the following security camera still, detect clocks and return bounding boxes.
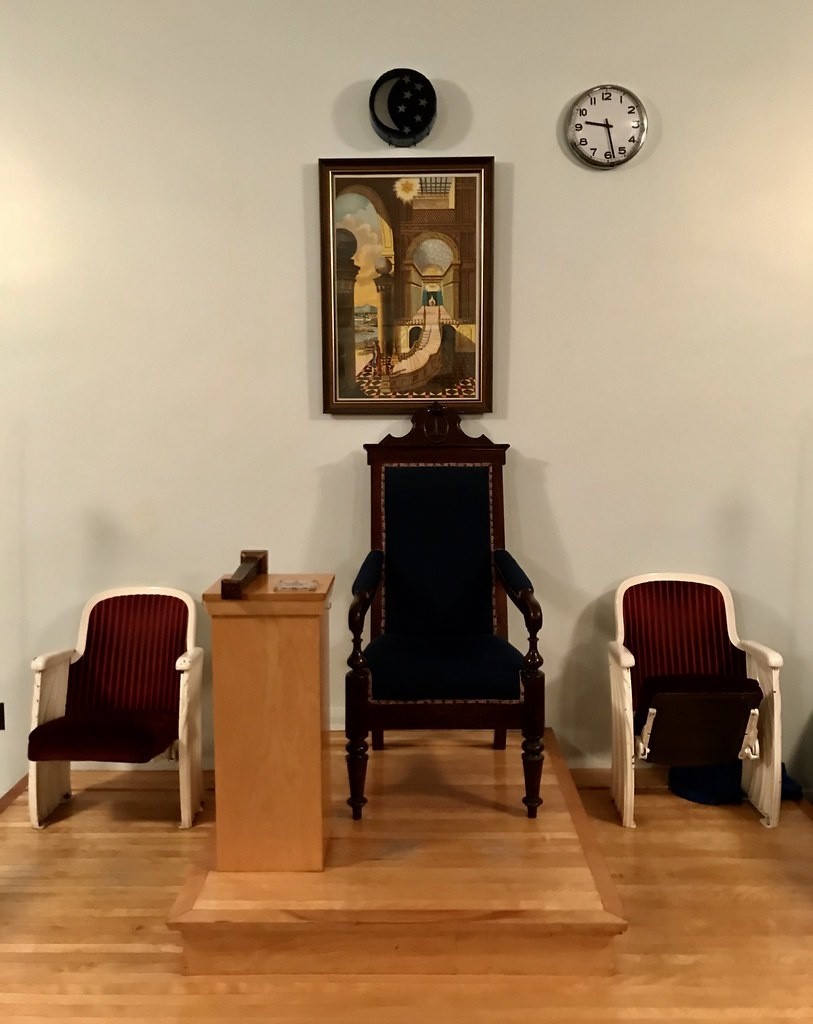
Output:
[564,85,648,171]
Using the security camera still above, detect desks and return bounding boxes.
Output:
[199,572,338,873]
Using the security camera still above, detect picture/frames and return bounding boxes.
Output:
[319,156,496,415]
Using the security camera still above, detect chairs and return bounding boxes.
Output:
[344,401,547,821]
[28,586,207,828]
[608,572,784,828]
